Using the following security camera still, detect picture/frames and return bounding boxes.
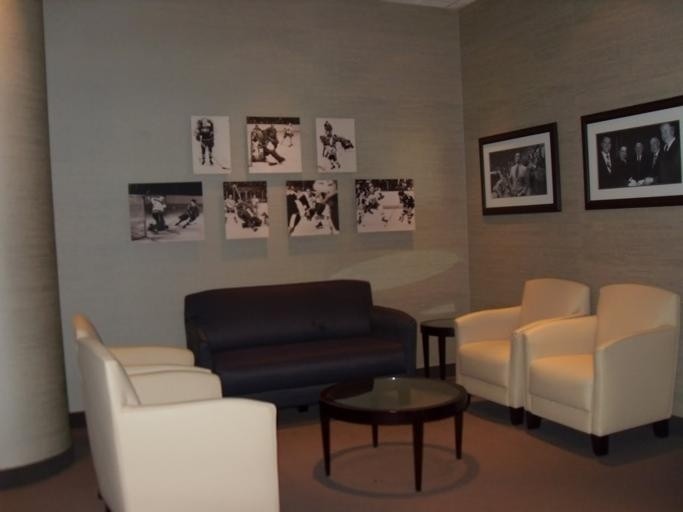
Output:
[581,95,683,209]
[479,122,561,215]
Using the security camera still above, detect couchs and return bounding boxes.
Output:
[184,280,417,412]
[455,278,591,425]
[73,313,212,499]
[524,283,680,456]
[80,338,280,512]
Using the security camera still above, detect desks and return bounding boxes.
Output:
[421,320,455,380]
